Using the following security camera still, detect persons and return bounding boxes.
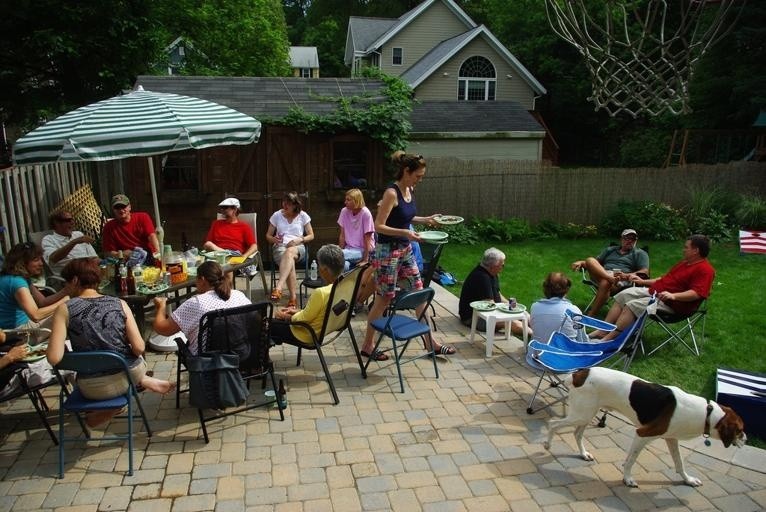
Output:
[459,248,532,340]
[361,150,455,361]
[46,256,179,427]
[101,194,162,271]
[41,211,102,277]
[266,191,315,307]
[204,197,257,287]
[587,236,714,343]
[0,242,71,345]
[268,242,346,350]
[337,189,375,271]
[571,227,651,318]
[153,262,262,362]
[351,199,423,318]
[0,328,77,399]
[525,272,590,376]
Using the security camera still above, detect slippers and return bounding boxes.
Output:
[361,348,389,360]
[428,345,456,356]
[287,300,297,307]
[271,289,281,300]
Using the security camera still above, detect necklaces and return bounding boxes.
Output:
[397,183,409,202]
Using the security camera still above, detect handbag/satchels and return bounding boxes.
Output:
[186,350,250,411]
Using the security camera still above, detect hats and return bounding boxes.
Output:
[218,197,241,209]
[621,229,638,237]
[110,194,130,206]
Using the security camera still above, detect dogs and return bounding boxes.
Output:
[543,367,748,488]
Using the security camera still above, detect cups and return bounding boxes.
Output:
[142,257,188,290]
[509,297,516,310]
[163,244,173,269]
[613,269,621,277]
[265,390,277,407]
[278,232,283,243]
[216,253,226,264]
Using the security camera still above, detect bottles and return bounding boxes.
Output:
[277,377,288,408]
[134,263,144,290]
[311,259,318,280]
[114,251,134,296]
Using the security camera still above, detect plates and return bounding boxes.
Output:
[469,300,498,310]
[135,284,169,294]
[205,251,229,259]
[498,302,527,312]
[18,346,49,362]
[418,231,450,244]
[433,215,463,225]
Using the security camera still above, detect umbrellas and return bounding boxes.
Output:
[10,84,262,253]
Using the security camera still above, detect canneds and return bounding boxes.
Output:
[509,298,517,311]
